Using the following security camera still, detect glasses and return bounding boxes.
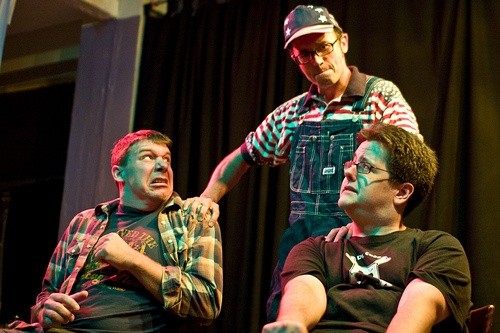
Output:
[342,160,405,183]
[295,37,341,65]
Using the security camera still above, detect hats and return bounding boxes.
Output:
[283,4,342,48]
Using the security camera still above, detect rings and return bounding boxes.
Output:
[342,226,348,230]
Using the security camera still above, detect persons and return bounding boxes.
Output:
[0,130,223,333]
[183,4,423,333]
[262,124,473,333]
